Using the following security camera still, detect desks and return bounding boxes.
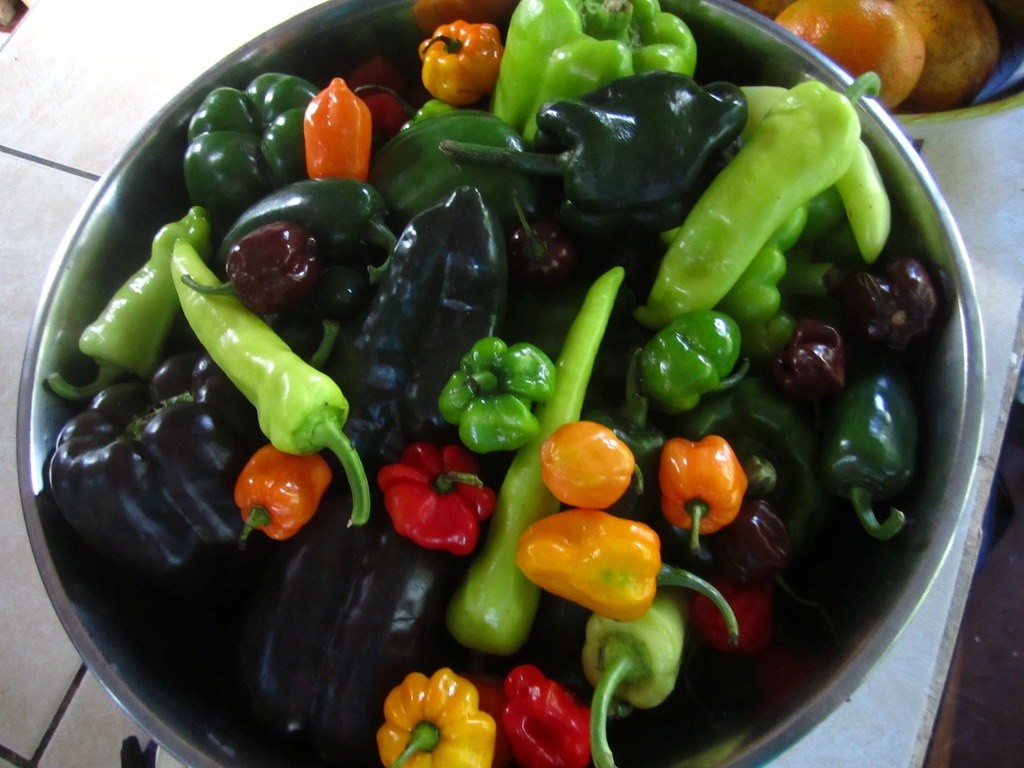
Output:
[3,1,1024,767]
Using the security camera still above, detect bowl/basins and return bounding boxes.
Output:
[17,0,989,768]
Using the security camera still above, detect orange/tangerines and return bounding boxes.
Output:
[735,1,1003,114]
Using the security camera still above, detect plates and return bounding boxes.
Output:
[881,0,1024,124]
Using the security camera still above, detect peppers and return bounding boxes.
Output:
[41,0,935,768]
